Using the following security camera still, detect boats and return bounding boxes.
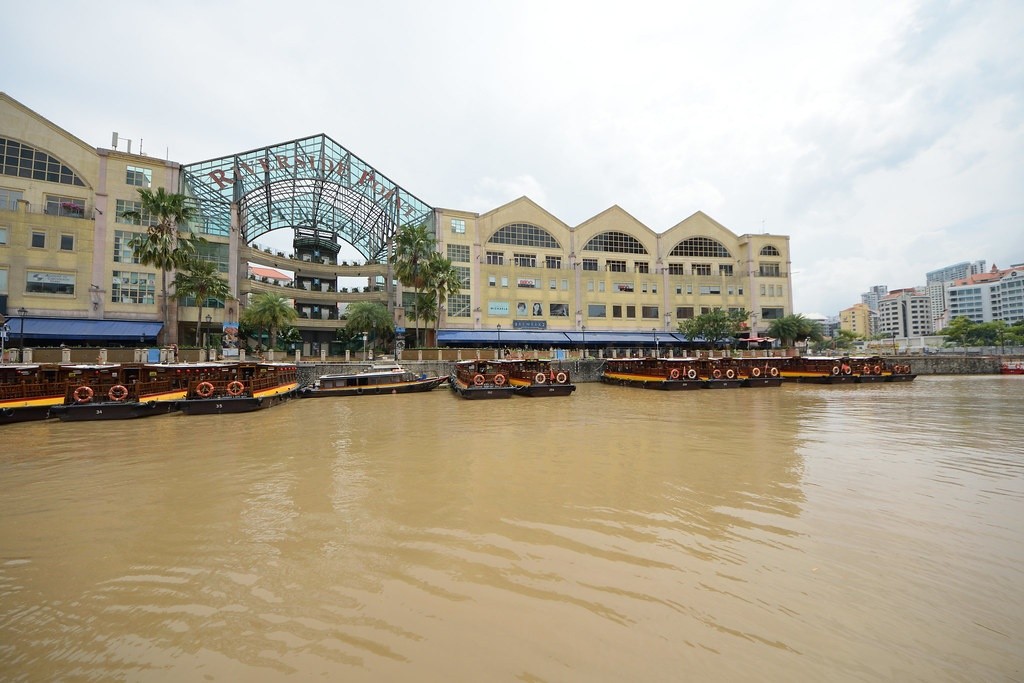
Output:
[447,359,518,400]
[597,359,917,391]
[497,359,577,397]
[45,363,190,423]
[298,365,450,398]
[175,361,303,417]
[0,363,74,429]
[999,360,1024,374]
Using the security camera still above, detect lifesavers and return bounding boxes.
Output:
[374,387,382,393]
[494,374,505,385]
[894,365,910,374]
[771,367,778,376]
[226,381,244,396]
[74,386,93,403]
[726,369,734,379]
[535,373,546,383]
[688,369,697,379]
[753,368,761,377]
[833,367,840,375]
[557,373,566,383]
[196,382,214,397]
[109,385,128,401]
[356,387,363,394]
[670,369,679,379]
[473,374,486,385]
[713,370,722,378]
[845,366,851,374]
[864,366,881,374]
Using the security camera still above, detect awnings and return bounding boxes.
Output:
[672,334,736,343]
[564,333,680,345]
[2,318,163,340]
[437,331,570,344]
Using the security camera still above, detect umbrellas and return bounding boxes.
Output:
[58,202,80,210]
[738,338,764,350]
[758,337,776,349]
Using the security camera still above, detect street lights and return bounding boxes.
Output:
[392,321,399,361]
[17,307,28,363]
[496,324,501,360]
[652,328,657,359]
[205,314,212,361]
[582,325,585,359]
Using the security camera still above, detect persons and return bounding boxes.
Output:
[533,303,542,316]
[172,344,180,363]
[505,348,511,360]
[517,302,527,315]
